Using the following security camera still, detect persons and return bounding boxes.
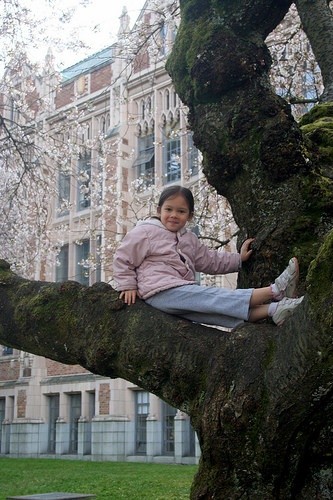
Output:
[114,186,306,327]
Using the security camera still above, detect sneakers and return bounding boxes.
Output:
[271,295,306,326]
[275,257,299,300]
